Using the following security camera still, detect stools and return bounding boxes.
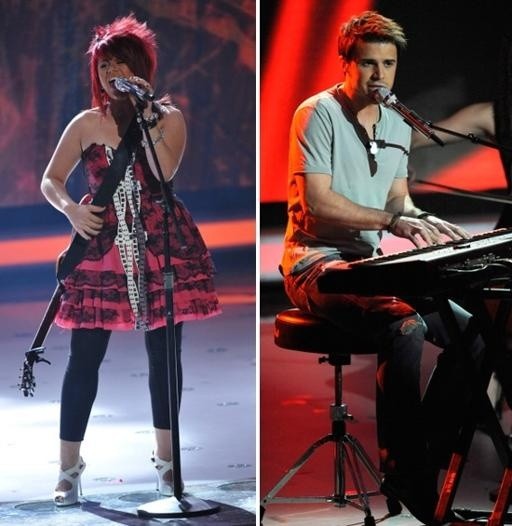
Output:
[260,307,403,526]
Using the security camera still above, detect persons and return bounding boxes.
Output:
[282,8,493,526]
[40,9,222,509]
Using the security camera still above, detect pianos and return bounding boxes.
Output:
[316,226,512,298]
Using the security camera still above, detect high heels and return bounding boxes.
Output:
[54,456,86,506]
[152,450,184,495]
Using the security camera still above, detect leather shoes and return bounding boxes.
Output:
[383,478,439,523]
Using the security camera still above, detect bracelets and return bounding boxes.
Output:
[141,130,164,149]
[140,119,158,130]
[417,211,437,219]
[137,111,159,124]
[386,212,404,231]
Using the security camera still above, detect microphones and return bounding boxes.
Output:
[114,77,155,102]
[374,88,444,146]
